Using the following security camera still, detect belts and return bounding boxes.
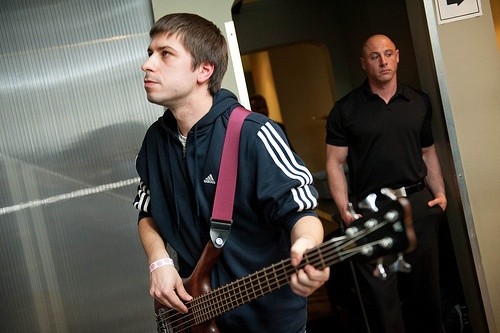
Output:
[393,180,425,198]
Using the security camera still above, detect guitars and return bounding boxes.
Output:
[153,187,414,333]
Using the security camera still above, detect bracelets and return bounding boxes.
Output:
[146,258,174,277]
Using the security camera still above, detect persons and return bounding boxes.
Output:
[325,32,447,333]
[134,13,331,333]
[249,94,295,152]
